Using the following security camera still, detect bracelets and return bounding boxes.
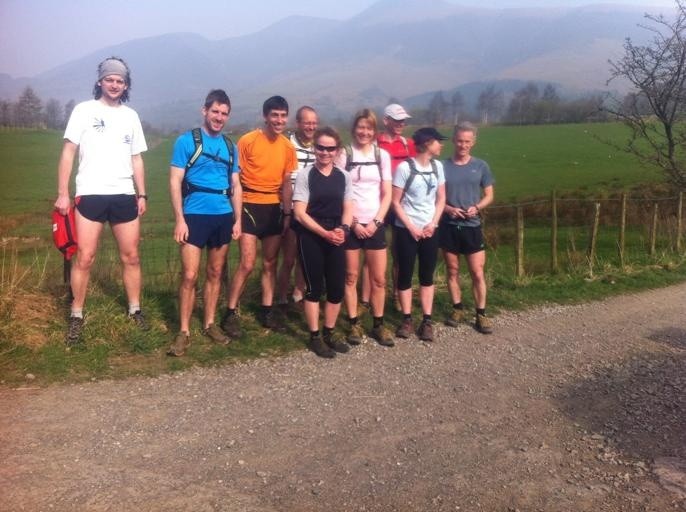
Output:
[137,194,147,200]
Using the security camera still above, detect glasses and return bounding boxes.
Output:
[315,143,338,151]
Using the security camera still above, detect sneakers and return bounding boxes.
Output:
[476,313,492,332]
[127,311,149,329]
[202,316,241,344]
[167,333,190,356]
[258,302,305,332]
[311,318,433,357]
[64,317,83,343]
[447,308,463,326]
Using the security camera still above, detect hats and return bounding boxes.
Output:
[413,128,447,143]
[384,104,411,120]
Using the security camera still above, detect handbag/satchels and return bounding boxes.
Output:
[52,207,78,260]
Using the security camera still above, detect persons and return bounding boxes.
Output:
[441,121,494,334]
[220,96,298,338]
[167,90,243,356]
[278,103,446,356]
[54,57,150,345]
